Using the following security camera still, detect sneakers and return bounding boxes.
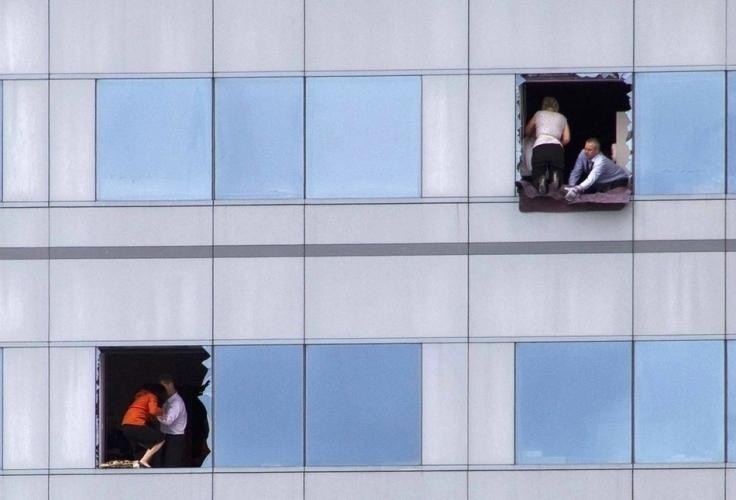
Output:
[551,170,560,189]
[538,175,546,193]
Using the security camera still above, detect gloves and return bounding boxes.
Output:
[564,186,579,201]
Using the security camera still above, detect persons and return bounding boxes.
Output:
[524,95,571,193]
[563,137,629,201]
[522,131,549,181]
[121,384,169,468]
[153,373,188,467]
